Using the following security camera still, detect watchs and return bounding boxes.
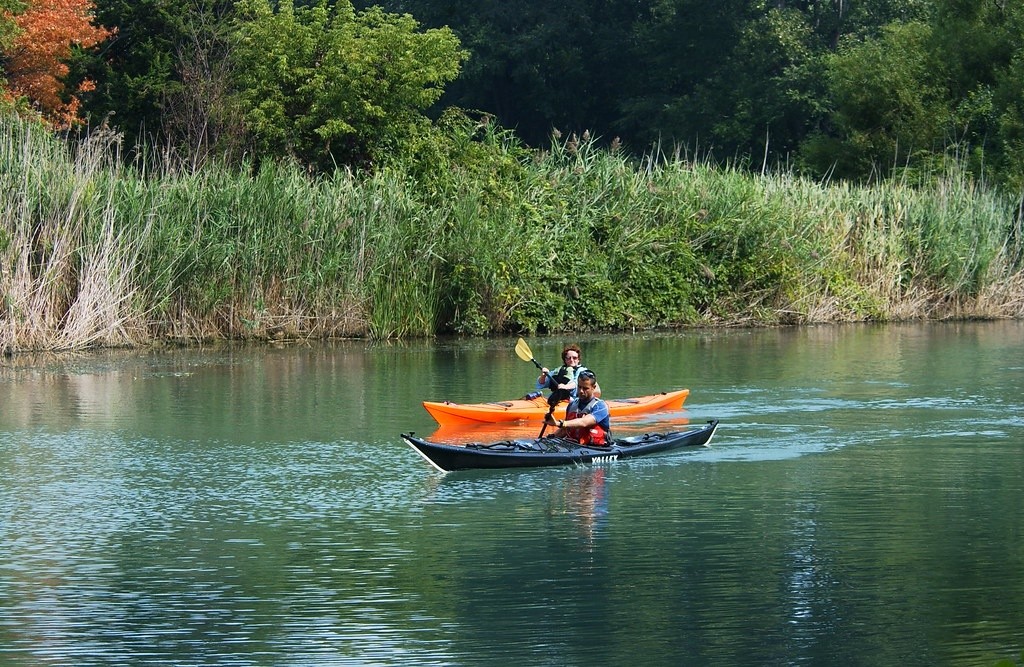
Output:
[558,419,564,428]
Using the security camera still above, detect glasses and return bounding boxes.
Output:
[579,371,596,381]
[565,356,579,360]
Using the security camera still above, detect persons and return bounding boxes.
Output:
[537,346,601,402]
[542,370,609,446]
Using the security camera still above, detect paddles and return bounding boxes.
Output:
[616,429,680,445]
[466,438,548,451]
[515,337,559,387]
[538,388,570,438]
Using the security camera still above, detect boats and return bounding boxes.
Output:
[422,388,690,428]
[401,417,720,472]
